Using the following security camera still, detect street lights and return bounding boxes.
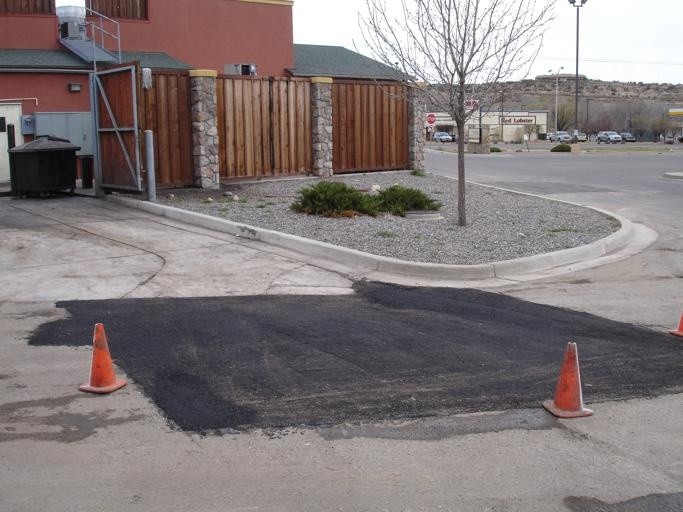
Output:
[548,66,564,131]
[568,0,588,135]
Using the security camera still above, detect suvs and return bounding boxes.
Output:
[596,131,622,144]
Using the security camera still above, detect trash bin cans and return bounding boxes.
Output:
[538,134,547,140]
[7,136,82,199]
[81,155,94,188]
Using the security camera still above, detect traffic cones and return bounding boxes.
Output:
[668,311,683,338]
[79,322,128,395]
[542,339,594,419]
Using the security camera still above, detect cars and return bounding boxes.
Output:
[549,131,572,142]
[620,133,636,142]
[434,131,453,142]
